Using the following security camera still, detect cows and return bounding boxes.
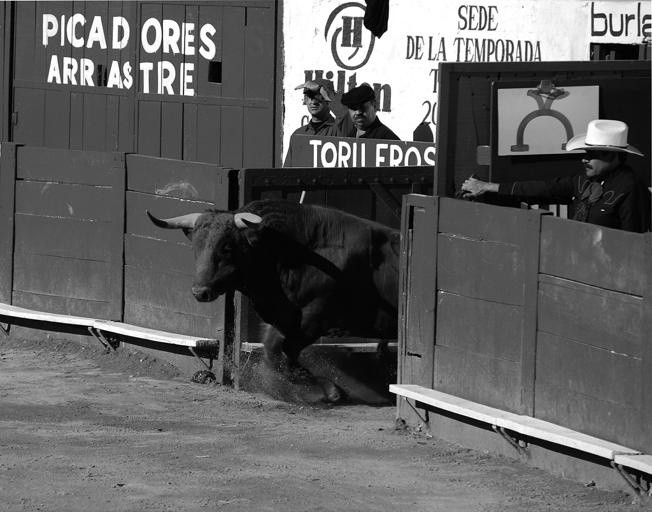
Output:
[143,196,401,411]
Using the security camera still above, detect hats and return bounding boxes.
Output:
[565,118,646,159]
[341,85,375,105]
[305,78,334,93]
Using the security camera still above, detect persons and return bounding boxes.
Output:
[461,120,645,230]
[341,84,399,141]
[283,79,353,167]
